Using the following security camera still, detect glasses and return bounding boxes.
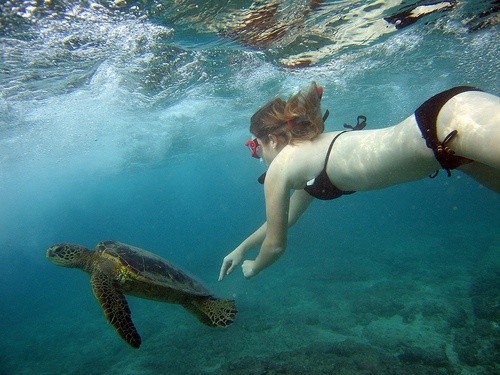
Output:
[243,137,263,160]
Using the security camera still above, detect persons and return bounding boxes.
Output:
[218,81,500,280]
